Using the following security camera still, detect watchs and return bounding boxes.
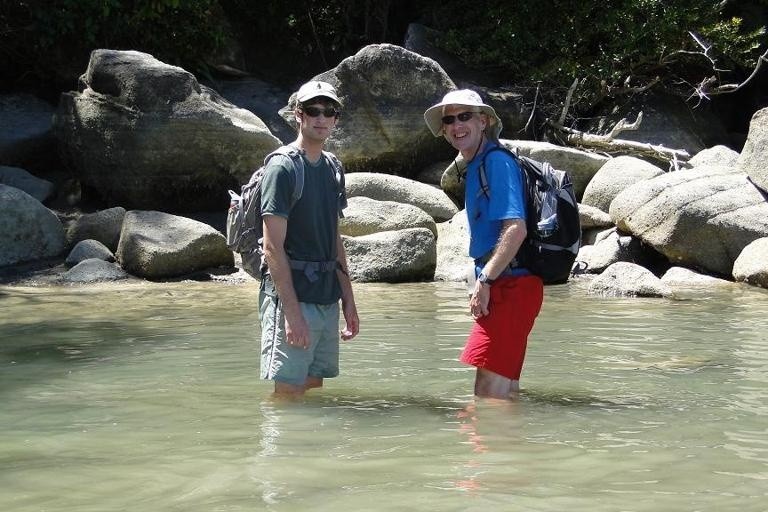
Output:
[479,273,494,286]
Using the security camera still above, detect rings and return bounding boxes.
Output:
[474,311,480,315]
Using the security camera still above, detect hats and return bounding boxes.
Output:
[295,81,345,106]
[423,89,502,138]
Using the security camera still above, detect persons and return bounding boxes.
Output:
[259,80,359,394]
[424,89,544,400]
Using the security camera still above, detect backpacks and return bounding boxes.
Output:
[224,166,271,281]
[518,154,582,286]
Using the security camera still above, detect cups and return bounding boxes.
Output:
[537,221,554,238]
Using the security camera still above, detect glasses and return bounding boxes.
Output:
[440,112,475,125]
[299,107,341,118]
